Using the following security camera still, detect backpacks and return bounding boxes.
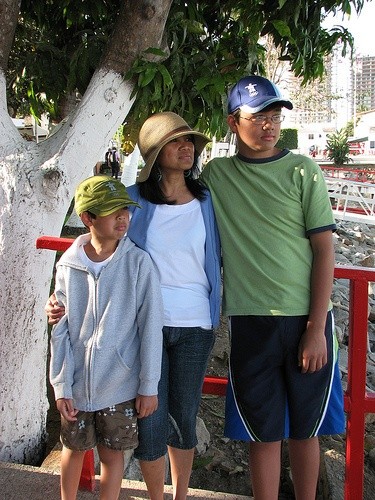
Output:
[109,151,117,163]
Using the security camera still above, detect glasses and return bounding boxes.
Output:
[239,115,286,125]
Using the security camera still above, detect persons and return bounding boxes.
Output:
[201,74,337,500]
[46,110,222,500]
[104,147,120,179]
[46,175,163,500]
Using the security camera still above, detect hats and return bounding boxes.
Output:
[136,111,213,182]
[75,175,142,217]
[227,75,293,115]
